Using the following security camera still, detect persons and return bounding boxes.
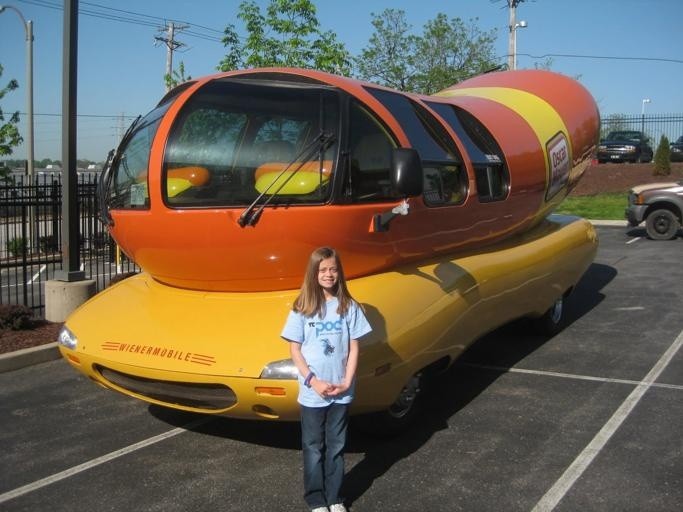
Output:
[279,247,373,512]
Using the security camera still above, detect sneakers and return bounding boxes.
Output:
[312,506,329,512]
[329,503,347,512]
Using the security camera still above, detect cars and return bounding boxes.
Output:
[669,135,682,161]
[597,130,654,164]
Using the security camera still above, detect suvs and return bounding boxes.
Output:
[624,177,683,241]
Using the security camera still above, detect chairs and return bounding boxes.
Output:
[249,139,294,181]
[352,133,393,179]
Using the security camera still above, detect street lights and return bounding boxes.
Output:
[641,98,652,138]
[513,20,528,70]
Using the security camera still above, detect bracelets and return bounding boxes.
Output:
[303,371,312,386]
